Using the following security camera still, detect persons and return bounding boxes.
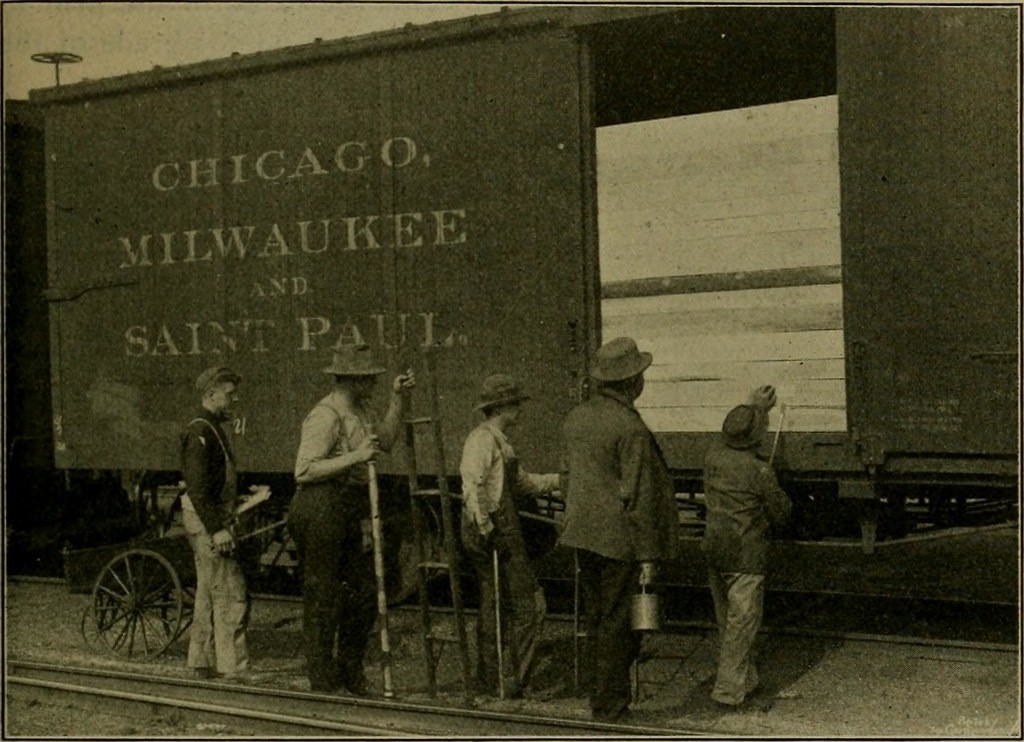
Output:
[460,375,561,698]
[287,343,416,696]
[703,384,793,712]
[177,366,276,683]
[562,336,681,722]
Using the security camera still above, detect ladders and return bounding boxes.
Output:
[398,347,474,694]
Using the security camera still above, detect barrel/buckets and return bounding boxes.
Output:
[628,581,661,632]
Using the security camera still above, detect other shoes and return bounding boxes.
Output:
[193,666,223,679]
[517,683,552,701]
[593,709,651,723]
[716,686,771,712]
[310,684,356,699]
[472,684,501,700]
[223,671,276,686]
[344,676,383,696]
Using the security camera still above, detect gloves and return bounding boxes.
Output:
[481,532,510,560]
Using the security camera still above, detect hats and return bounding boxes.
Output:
[591,337,653,382]
[196,366,238,395]
[327,342,386,376]
[472,374,531,413]
[722,404,769,449]
